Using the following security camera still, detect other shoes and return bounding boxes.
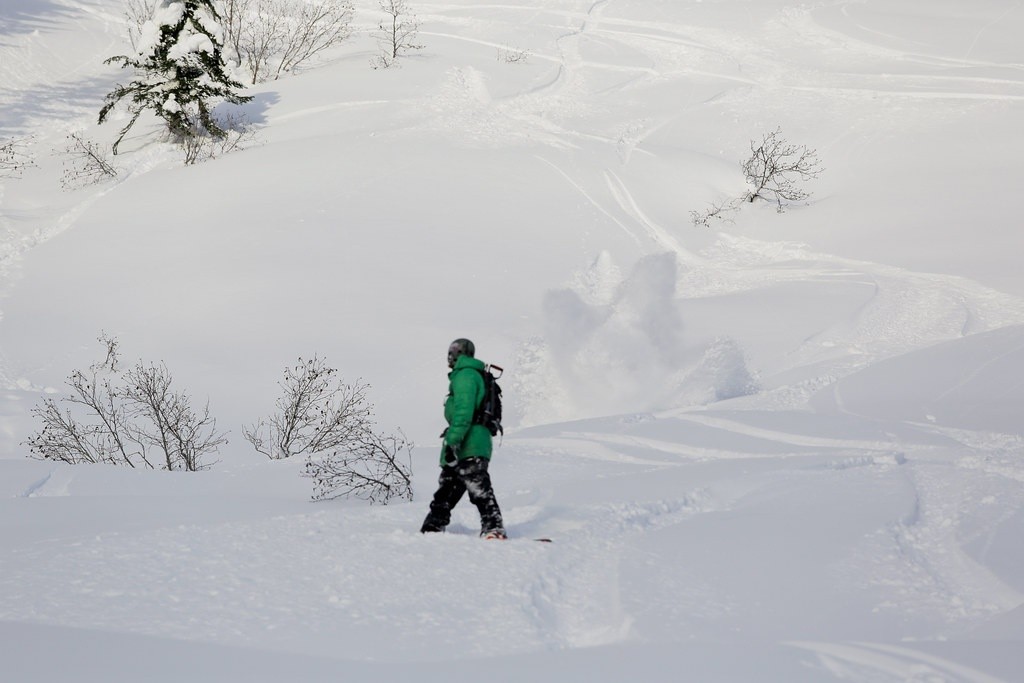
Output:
[479,528,508,540]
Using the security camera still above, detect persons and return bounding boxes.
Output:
[421,339,506,539]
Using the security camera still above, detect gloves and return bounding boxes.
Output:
[445,443,458,466]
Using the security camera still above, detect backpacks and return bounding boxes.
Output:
[475,368,502,436]
